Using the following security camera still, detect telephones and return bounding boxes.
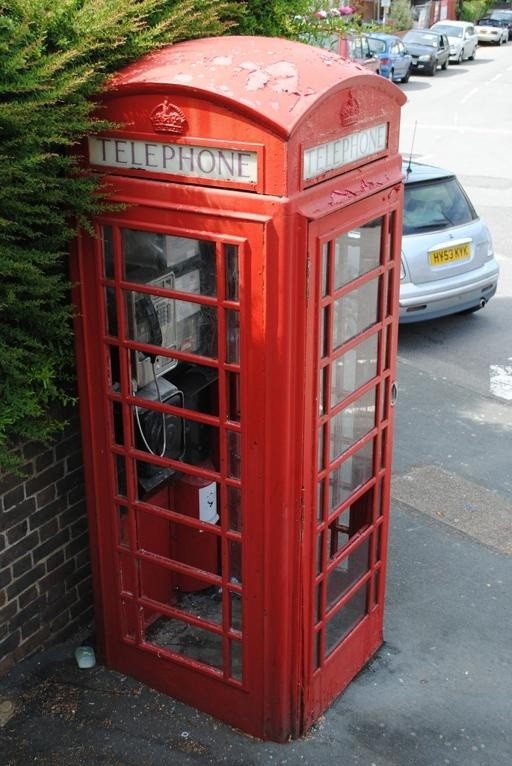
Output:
[107,271,180,392]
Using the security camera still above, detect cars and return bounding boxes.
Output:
[324,151,501,338]
[299,8,512,85]
